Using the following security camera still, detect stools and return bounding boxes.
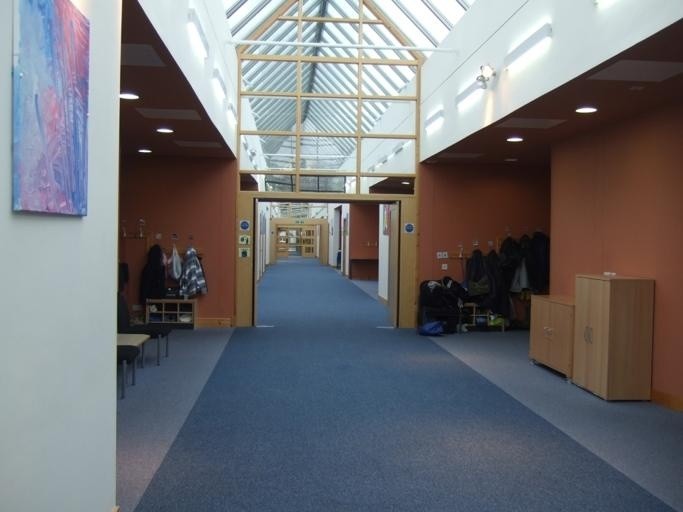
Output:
[116,345,140,399]
[120,323,172,367]
[117,331,151,371]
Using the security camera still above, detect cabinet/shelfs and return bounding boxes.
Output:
[572,273,654,404]
[457,302,496,328]
[530,291,574,381]
[145,298,199,331]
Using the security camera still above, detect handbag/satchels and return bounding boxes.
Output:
[419,321,443,336]
[169,247,182,280]
